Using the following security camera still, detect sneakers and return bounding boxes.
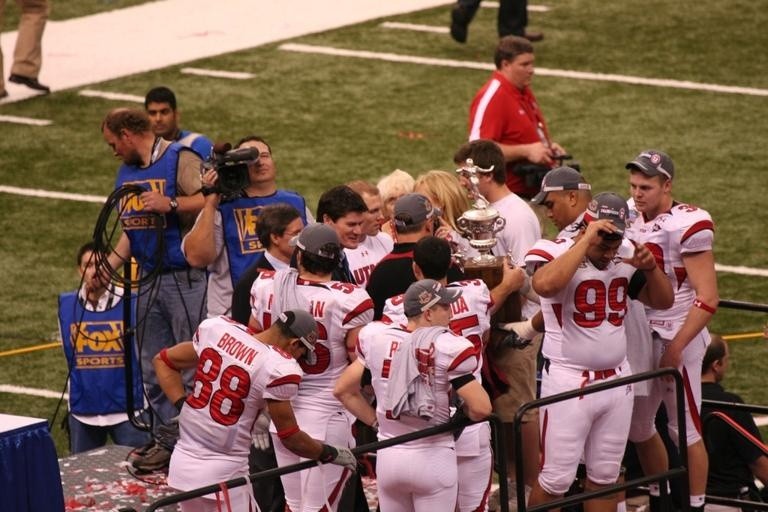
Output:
[483,477,533,512]
[133,445,171,472]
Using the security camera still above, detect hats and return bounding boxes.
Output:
[403,278,463,318]
[625,148,677,183]
[289,223,342,261]
[392,193,442,226]
[276,309,317,366]
[530,166,591,209]
[581,191,630,235]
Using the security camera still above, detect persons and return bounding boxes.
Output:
[450,0,544,43]
[0,0,50,99]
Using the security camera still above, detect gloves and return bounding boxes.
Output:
[323,443,357,474]
[498,318,539,350]
[250,409,271,450]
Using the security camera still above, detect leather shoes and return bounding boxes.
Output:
[501,31,544,42]
[451,3,468,45]
[8,73,50,94]
[0,88,8,99]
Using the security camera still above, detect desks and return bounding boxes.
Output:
[0,410,70,510]
